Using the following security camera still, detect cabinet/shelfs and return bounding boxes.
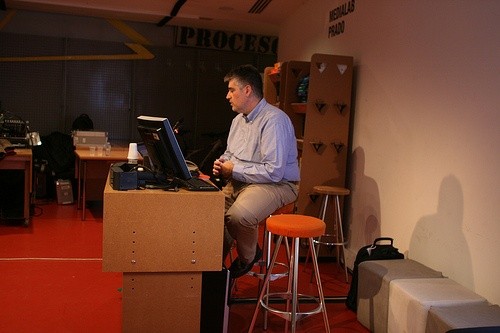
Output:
[260,65,284,112]
[281,52,354,261]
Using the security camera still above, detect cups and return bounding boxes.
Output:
[127,143,139,160]
[97,147,103,156]
[90,147,96,156]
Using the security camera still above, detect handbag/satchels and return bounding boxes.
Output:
[345,238,404,315]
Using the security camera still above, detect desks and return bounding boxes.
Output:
[74,147,144,220]
[0,147,32,226]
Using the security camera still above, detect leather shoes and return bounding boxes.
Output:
[228,243,263,278]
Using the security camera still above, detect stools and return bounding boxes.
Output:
[230,184,350,333]
[357,258,500,333]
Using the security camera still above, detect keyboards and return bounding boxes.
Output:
[177,179,219,192]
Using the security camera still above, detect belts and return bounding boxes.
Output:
[288,181,300,185]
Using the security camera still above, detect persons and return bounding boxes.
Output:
[212,64,301,278]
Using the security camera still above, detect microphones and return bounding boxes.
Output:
[173,117,186,133]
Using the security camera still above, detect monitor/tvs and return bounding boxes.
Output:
[137,115,192,189]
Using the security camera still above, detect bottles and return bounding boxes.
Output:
[105,142,111,157]
[297,76,308,103]
[128,159,137,164]
[25,120,30,139]
